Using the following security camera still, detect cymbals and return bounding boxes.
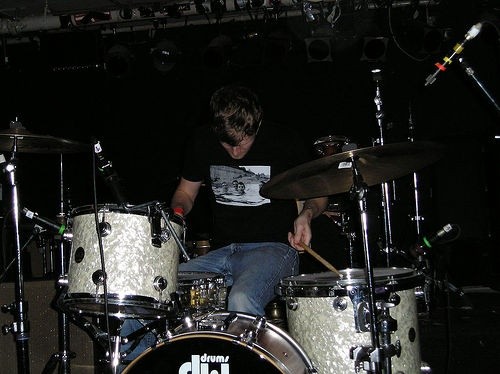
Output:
[258,139,440,199]
[0,129,91,154]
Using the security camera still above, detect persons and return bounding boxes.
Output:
[117,85,329,372]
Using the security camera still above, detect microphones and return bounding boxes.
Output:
[424,21,484,87]
[19,206,66,235]
[416,224,455,254]
[93,138,113,171]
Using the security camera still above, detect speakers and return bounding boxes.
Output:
[0,276,99,374]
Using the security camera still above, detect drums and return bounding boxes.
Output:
[118,311,316,374]
[177,271,227,313]
[57,202,185,317]
[277,265,426,374]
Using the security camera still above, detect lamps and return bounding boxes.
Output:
[104,28,389,79]
